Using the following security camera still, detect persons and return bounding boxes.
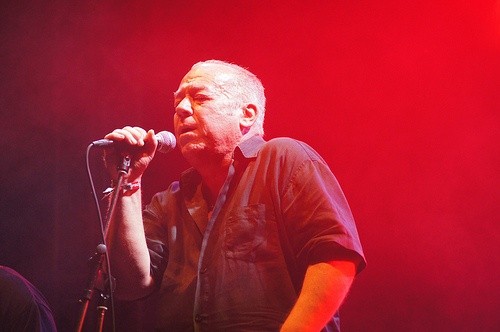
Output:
[103,58,366,332]
[0,265,58,332]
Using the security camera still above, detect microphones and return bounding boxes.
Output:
[93,131,176,153]
